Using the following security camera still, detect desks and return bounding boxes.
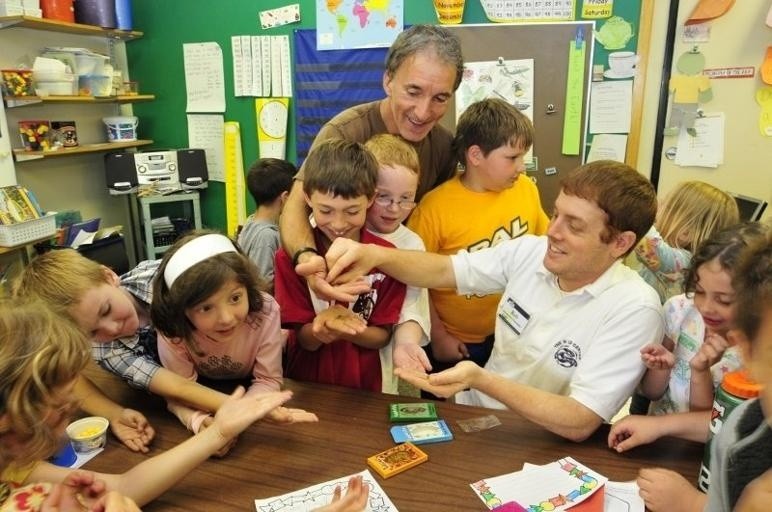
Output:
[0,234,66,268]
[68,375,714,512]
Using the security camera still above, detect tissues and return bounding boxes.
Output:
[32,57,79,95]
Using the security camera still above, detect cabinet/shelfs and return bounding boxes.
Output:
[138,189,203,262]
[1,16,154,164]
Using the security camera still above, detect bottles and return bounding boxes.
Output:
[697,367,760,495]
[47,437,77,467]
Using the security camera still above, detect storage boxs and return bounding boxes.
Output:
[78,72,113,98]
[38,46,111,75]
[1,0,43,20]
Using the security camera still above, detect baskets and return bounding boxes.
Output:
[0,211,58,248]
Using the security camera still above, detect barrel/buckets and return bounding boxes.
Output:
[102,116,139,142]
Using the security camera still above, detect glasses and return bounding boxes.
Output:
[374,197,417,210]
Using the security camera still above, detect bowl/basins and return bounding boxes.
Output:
[69,416,109,452]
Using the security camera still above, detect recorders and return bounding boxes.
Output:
[104,149,209,191]
